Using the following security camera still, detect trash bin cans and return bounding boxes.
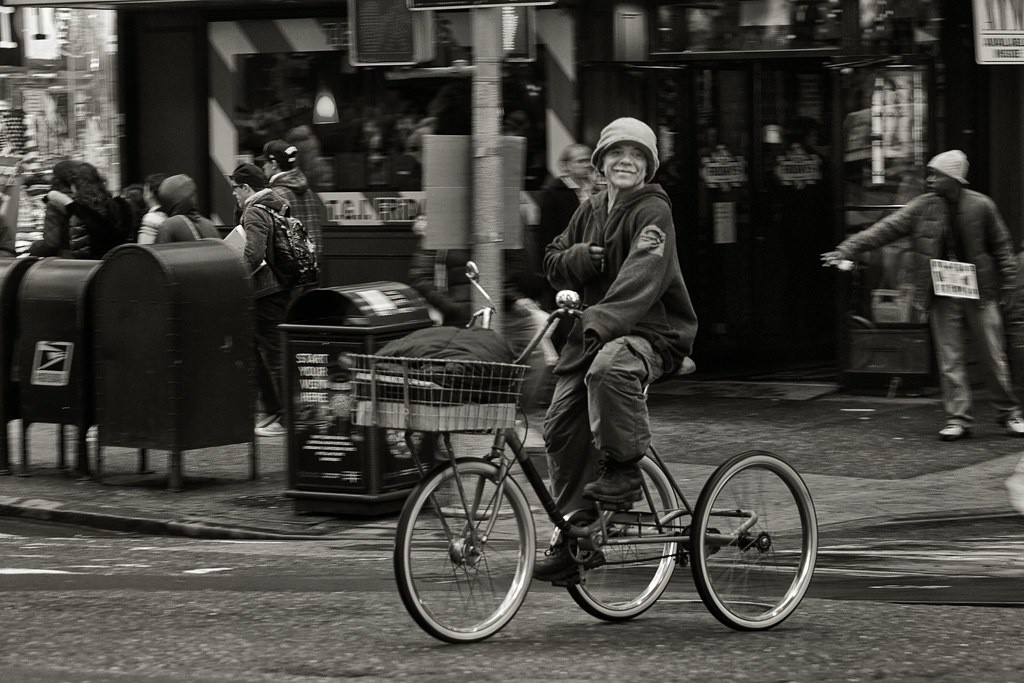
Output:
[280,281,441,520]
[89,238,259,497]
[0,251,102,478]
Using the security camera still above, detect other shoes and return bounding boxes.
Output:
[1007,418,1024,434]
[940,424,965,441]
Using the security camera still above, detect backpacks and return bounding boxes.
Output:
[253,204,322,288]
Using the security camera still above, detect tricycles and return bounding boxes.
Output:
[346,262,818,644]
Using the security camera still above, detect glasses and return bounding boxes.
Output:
[232,183,244,189]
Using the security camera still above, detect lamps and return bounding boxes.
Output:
[312,53,339,124]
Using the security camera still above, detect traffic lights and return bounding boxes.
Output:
[349,0,436,66]
[501,2,538,63]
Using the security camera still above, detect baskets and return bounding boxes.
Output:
[346,352,531,434]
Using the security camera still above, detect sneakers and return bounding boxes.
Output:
[529,541,606,582]
[585,453,647,504]
[254,422,288,437]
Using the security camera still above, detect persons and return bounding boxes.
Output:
[532,117,699,582]
[252,139,328,264]
[29,159,285,440]
[535,144,594,310]
[224,162,305,437]
[819,150,1024,442]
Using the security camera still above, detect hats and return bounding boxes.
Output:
[592,118,659,182]
[255,139,297,171]
[929,149,969,186]
[229,162,265,191]
